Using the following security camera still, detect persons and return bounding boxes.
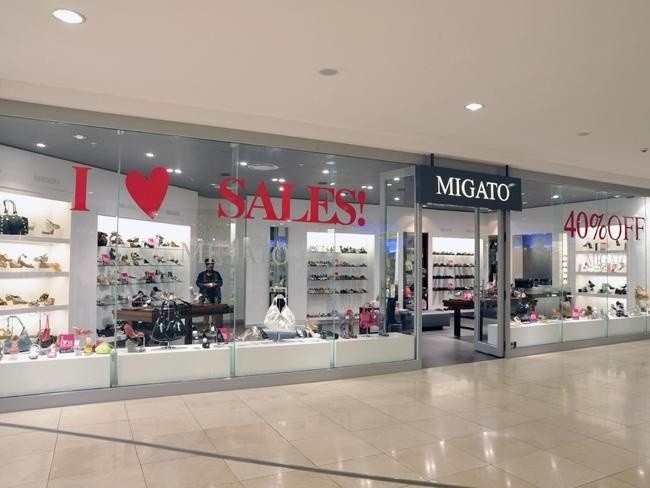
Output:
[196,258,224,334]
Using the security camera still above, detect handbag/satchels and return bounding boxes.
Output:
[264,296,295,331]
[152,301,189,342]
[0,199,28,235]
[41,318,51,341]
[360,303,381,327]
[59,334,74,352]
[3,315,33,354]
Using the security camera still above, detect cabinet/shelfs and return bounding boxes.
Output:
[305,230,373,324]
[94,215,191,333]
[432,248,475,316]
[0,184,69,353]
[575,223,647,312]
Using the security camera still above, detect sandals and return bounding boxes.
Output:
[97,231,185,302]
[0,252,49,268]
[1,293,55,306]
[307,261,367,266]
[310,245,367,253]
[42,218,61,233]
[84,337,93,354]
[74,336,81,354]
[47,344,57,358]
[310,274,368,280]
[309,288,366,293]
[29,344,41,358]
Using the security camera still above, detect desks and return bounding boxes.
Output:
[112,300,233,327]
[442,294,537,336]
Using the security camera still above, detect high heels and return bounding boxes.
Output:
[320,330,338,339]
[203,333,210,349]
[305,327,320,337]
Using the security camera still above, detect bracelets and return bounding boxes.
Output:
[212,282,215,287]
